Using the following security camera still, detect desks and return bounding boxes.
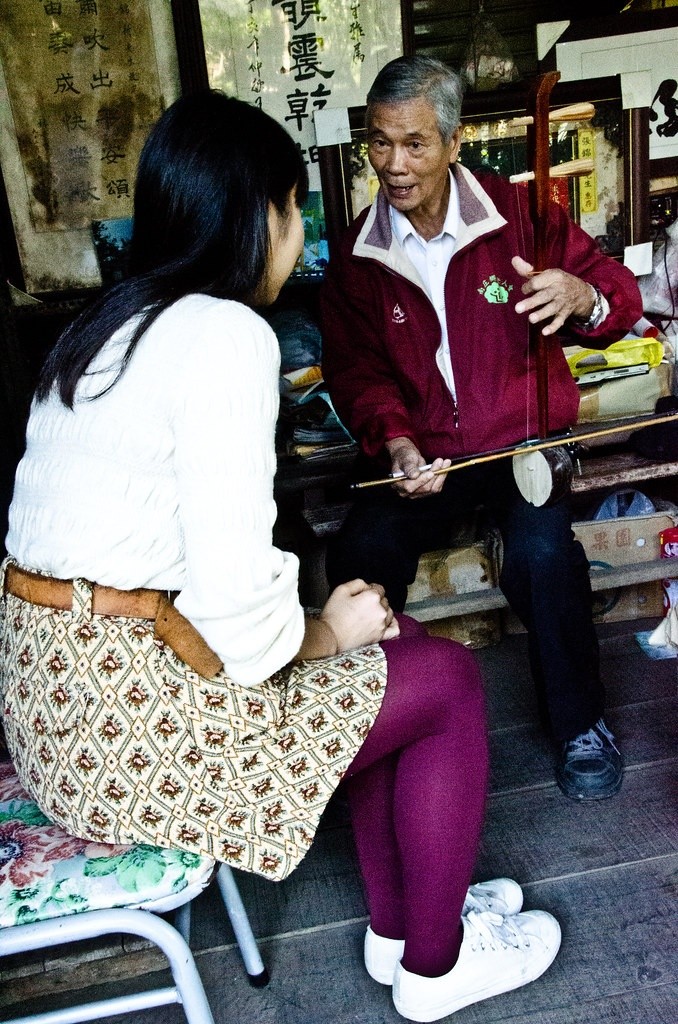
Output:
[273,409,678,611]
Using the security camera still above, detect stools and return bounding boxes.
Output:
[0,746,271,1024]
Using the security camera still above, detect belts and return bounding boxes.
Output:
[4,562,224,681]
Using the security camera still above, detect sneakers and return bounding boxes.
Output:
[393,910,561,1022]
[557,714,623,798]
[364,878,523,985]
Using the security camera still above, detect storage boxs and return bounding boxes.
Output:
[400,540,503,654]
[471,496,678,637]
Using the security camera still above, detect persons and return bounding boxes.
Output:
[0,88,564,1024]
[313,55,645,803]
